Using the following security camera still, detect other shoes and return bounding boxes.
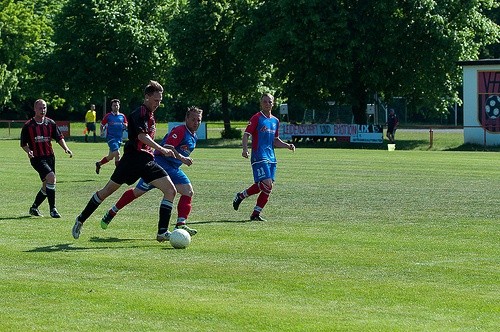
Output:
[84,137,97,142]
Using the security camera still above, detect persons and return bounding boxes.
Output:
[386,108,399,144]
[96,99,129,175]
[85,104,98,143]
[100,106,204,237]
[71,79,178,242]
[232,93,296,222]
[20,99,73,219]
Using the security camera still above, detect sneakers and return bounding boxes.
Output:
[233,193,241,211]
[72,218,83,238]
[250,215,268,221]
[100,211,113,230]
[29,208,43,217]
[173,225,197,236]
[156,231,174,241]
[50,208,60,218]
[96,163,101,174]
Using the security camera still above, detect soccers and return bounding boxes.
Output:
[169,229,192,248]
